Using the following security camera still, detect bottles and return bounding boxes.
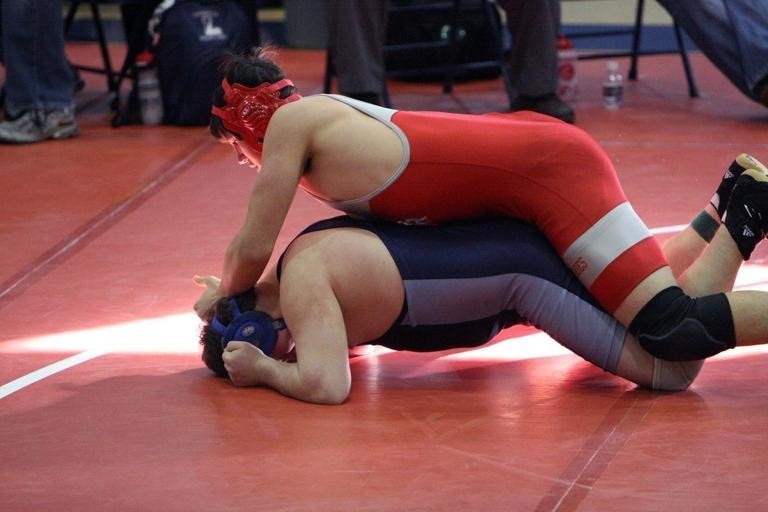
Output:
[135,52,164,125]
[555,35,578,110]
[602,60,624,111]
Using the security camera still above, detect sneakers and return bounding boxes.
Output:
[1,101,80,143]
[717,150,768,260]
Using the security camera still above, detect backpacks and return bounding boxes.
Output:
[120,8,225,128]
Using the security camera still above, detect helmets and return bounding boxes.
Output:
[212,78,300,154]
[205,301,280,367]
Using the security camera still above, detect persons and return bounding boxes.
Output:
[0,1,86,143]
[333,1,574,123]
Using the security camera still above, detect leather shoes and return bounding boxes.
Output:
[508,94,576,129]
